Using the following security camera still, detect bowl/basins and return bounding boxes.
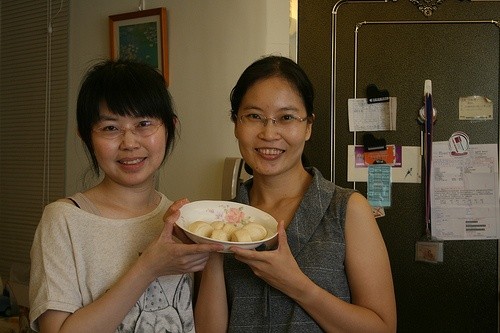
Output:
[175,199,279,253]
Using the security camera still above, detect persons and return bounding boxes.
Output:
[25,57,231,332]
[162,53,398,332]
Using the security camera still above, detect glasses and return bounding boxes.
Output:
[235,108,309,129]
[91,114,164,140]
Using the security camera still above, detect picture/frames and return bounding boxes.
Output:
[108,7,169,88]
